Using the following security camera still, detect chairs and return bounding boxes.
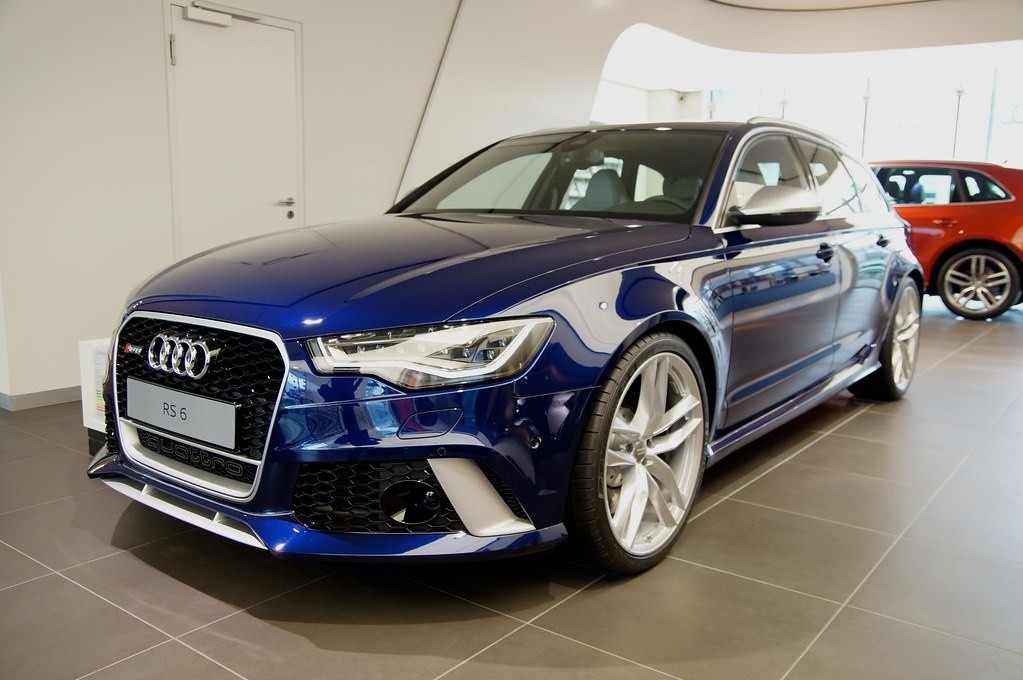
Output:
[661,172,703,208]
[569,169,632,211]
[908,183,925,204]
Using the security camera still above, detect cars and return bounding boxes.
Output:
[865,160,1023,319]
[88,116,925,579]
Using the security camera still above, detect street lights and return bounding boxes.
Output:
[780,87,788,119]
[707,90,716,120]
[862,77,870,158]
[952,84,965,160]
